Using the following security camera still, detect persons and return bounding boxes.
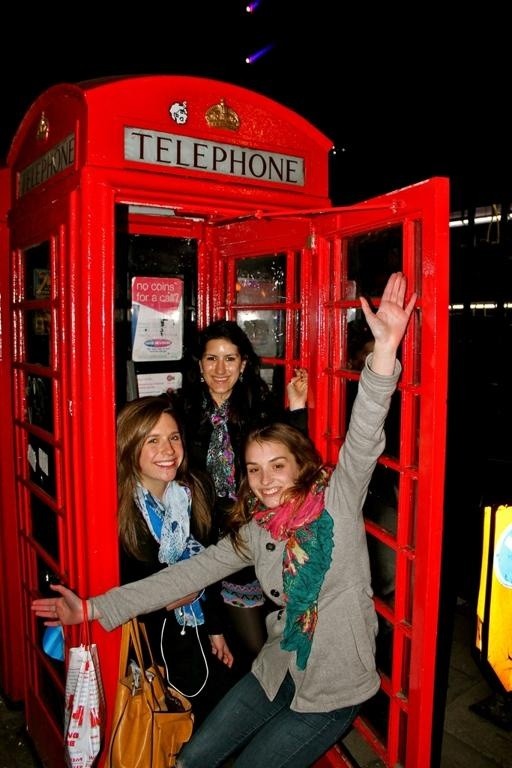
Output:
[31,271,418,768]
[347,317,398,636]
[117,397,258,737]
[177,320,308,686]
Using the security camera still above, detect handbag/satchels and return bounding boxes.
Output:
[64,599,106,768]
[104,617,195,768]
